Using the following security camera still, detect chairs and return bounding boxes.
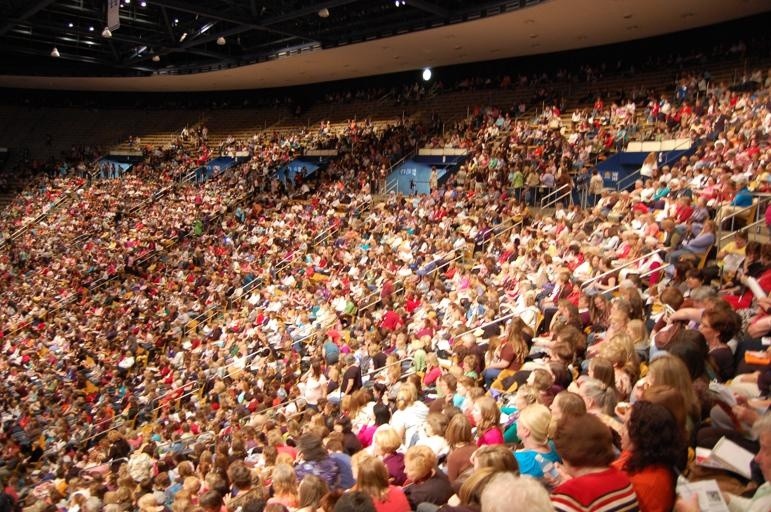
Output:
[112,91,492,157]
[494,68,674,138]
